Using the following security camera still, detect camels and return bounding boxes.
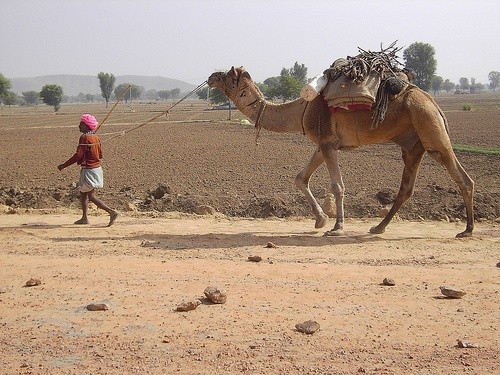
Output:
[208,52,474,238]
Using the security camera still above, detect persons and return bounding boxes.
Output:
[58,114,119,227]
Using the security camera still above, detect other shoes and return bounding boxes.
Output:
[108,209,121,226]
[74,217,88,224]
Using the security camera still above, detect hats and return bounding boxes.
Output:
[80,114,98,130]
